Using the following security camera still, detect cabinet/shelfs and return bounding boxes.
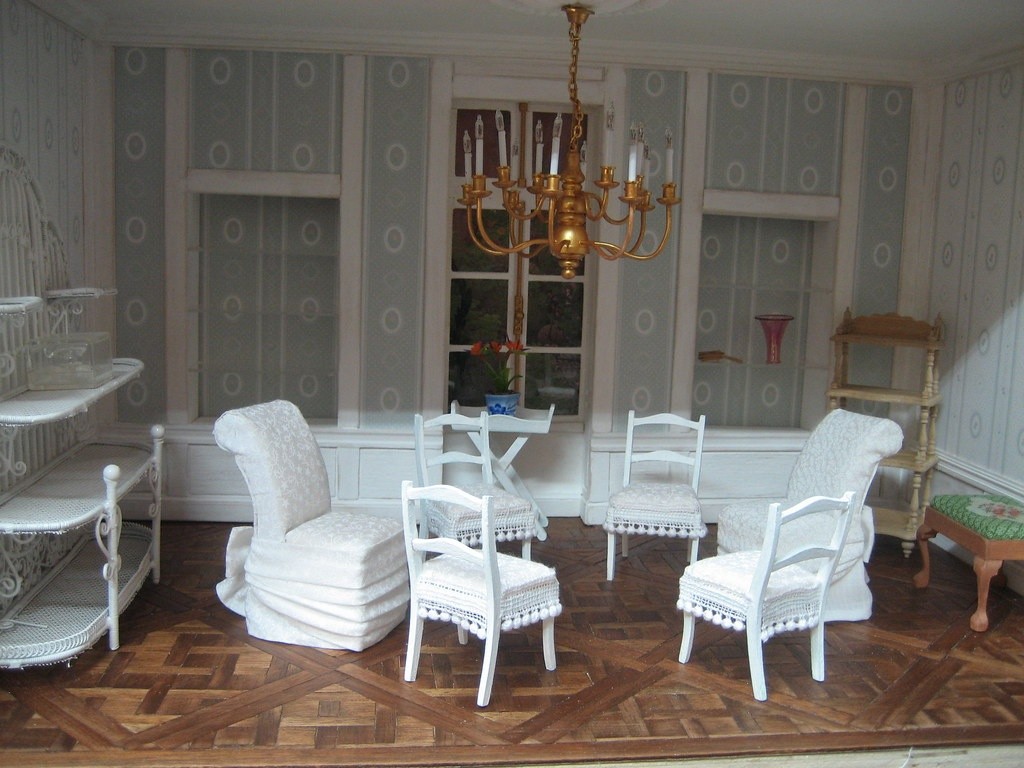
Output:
[688,189,842,439]
[185,165,360,433]
[0,140,166,670]
[824,306,946,559]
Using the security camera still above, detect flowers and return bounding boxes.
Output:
[470,337,531,393]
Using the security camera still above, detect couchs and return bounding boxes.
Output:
[211,398,412,652]
[717,407,905,623]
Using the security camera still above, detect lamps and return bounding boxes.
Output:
[457,0,682,281]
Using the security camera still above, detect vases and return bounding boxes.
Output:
[485,392,522,418]
[755,313,794,365]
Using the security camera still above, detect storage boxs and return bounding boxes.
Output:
[24,331,115,391]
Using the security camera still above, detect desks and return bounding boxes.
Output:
[449,399,556,541]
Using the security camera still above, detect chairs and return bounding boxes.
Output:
[677,492,857,701]
[399,480,562,707]
[602,410,706,582]
[412,411,536,562]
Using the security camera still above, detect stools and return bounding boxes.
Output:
[914,495,1024,632]
